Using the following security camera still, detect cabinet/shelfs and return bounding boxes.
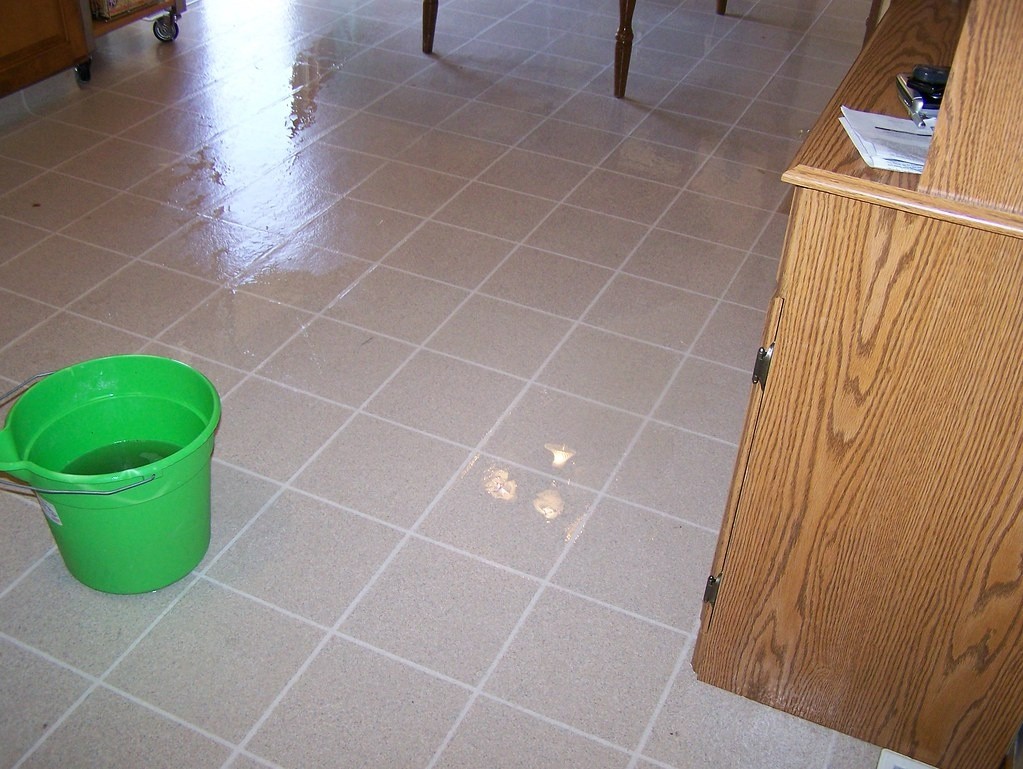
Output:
[689,2,1023,769]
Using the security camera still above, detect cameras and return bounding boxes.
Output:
[896,65,954,129]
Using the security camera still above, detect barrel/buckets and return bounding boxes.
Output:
[0,354,222,594]
